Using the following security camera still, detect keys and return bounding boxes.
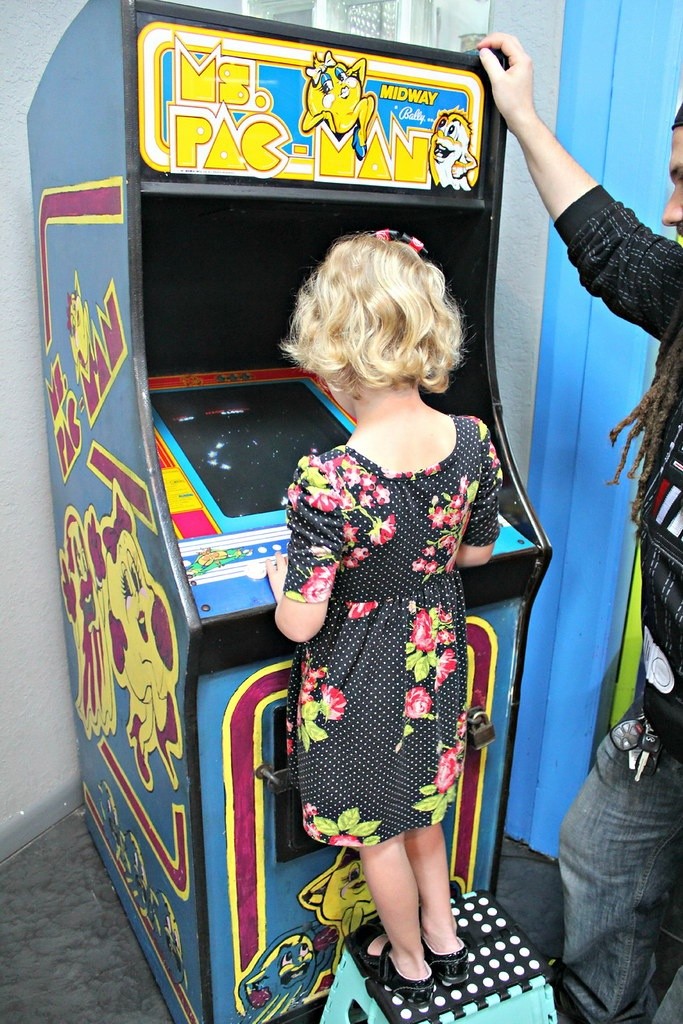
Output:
[611,709,664,783]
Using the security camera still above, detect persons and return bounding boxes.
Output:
[474,31,683,1024]
[267,231,509,1010]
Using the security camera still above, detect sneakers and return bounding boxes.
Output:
[541,954,590,1024]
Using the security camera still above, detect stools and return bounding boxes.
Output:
[319,890,559,1024]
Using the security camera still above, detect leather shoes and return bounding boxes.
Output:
[351,922,433,1006]
[419,934,471,986]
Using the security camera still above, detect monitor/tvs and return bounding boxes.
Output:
[148,382,351,518]
